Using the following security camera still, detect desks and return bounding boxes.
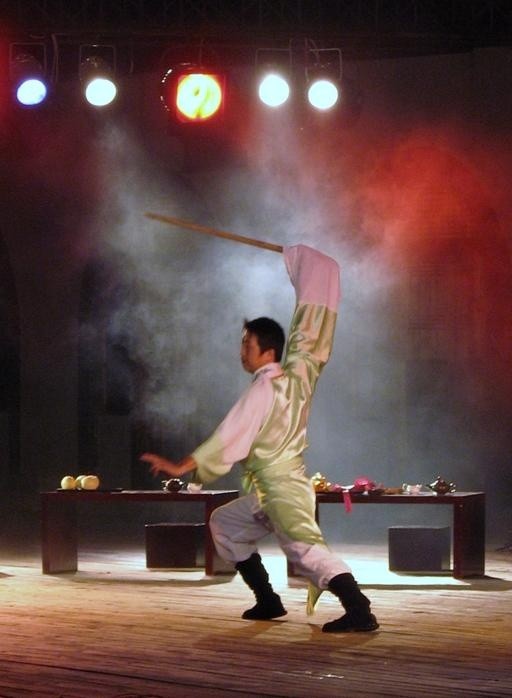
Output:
[287,491,486,579]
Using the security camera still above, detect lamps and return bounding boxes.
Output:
[304,39,343,113]
[249,41,293,109]
[79,42,133,109]
[10,35,60,108]
[158,36,229,121]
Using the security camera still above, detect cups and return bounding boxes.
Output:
[187,482,203,493]
[406,482,420,494]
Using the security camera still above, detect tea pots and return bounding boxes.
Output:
[310,472,328,493]
[426,476,456,495]
[161,478,186,492]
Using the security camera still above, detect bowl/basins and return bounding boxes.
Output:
[367,488,404,495]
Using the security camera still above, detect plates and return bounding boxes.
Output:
[57,486,126,494]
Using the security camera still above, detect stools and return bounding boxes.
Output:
[40,487,239,575]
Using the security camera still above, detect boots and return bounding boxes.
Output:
[323,571,379,632]
[235,552,287,620]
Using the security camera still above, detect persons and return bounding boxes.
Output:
[138,244,381,635]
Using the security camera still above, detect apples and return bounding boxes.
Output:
[61,474,100,490]
